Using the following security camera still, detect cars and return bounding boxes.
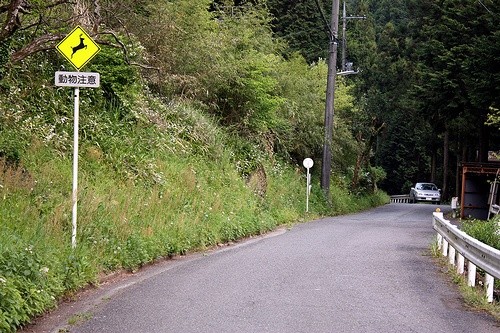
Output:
[409,183,441,204]
